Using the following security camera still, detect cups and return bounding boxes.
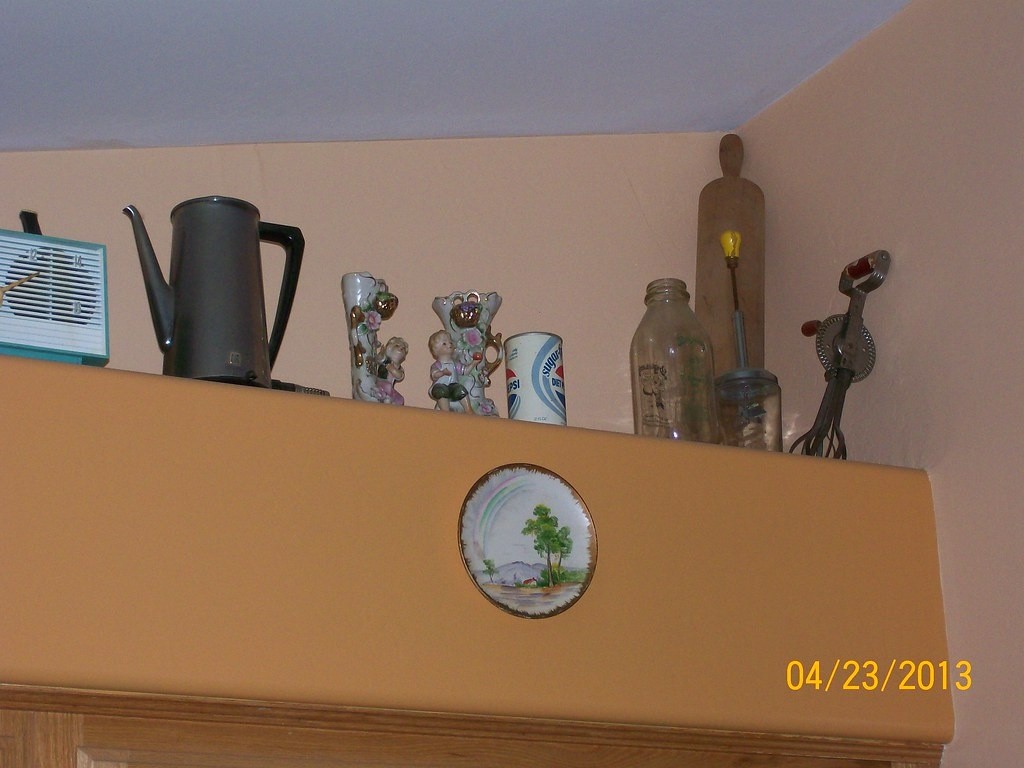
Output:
[503,331,566,424]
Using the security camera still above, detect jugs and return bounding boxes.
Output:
[124,195,305,388]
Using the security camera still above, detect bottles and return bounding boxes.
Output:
[629,278,714,444]
